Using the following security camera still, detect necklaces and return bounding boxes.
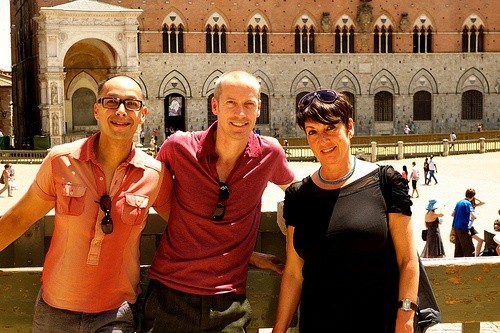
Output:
[319,155,355,184]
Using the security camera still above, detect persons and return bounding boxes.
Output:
[141,70,301,333]
[0,163,14,197]
[403,122,416,135]
[274,128,280,138]
[283,139,289,154]
[165,126,179,138]
[450,187,500,256]
[476,121,483,131]
[146,128,160,156]
[409,161,419,198]
[401,165,408,181]
[139,130,145,145]
[449,132,456,150]
[269,89,420,333]
[421,198,447,258]
[253,128,261,135]
[0,74,283,333]
[423,154,438,185]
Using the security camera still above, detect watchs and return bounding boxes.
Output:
[398,298,420,315]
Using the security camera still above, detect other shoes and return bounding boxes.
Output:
[8,195,13,197]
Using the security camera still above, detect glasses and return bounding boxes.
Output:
[210,181,232,221]
[94,194,113,234]
[297,89,342,113]
[97,96,144,111]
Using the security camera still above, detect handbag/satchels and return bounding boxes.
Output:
[449,227,457,243]
[422,229,428,241]
[0,172,5,184]
[413,250,442,333]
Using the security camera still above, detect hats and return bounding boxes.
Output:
[425,199,439,211]
